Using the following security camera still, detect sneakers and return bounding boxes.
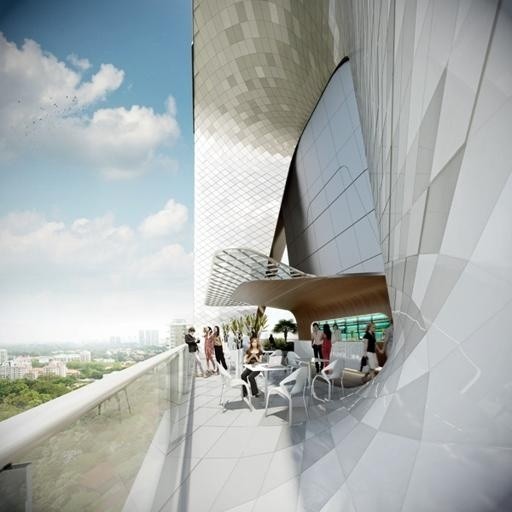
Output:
[195,369,219,379]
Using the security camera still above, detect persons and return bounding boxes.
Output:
[332,323,341,334]
[310,323,326,373]
[185,327,204,378]
[240,337,263,398]
[320,324,331,369]
[359,322,383,372]
[380,318,393,357]
[268,334,275,343]
[213,326,228,369]
[201,326,216,373]
